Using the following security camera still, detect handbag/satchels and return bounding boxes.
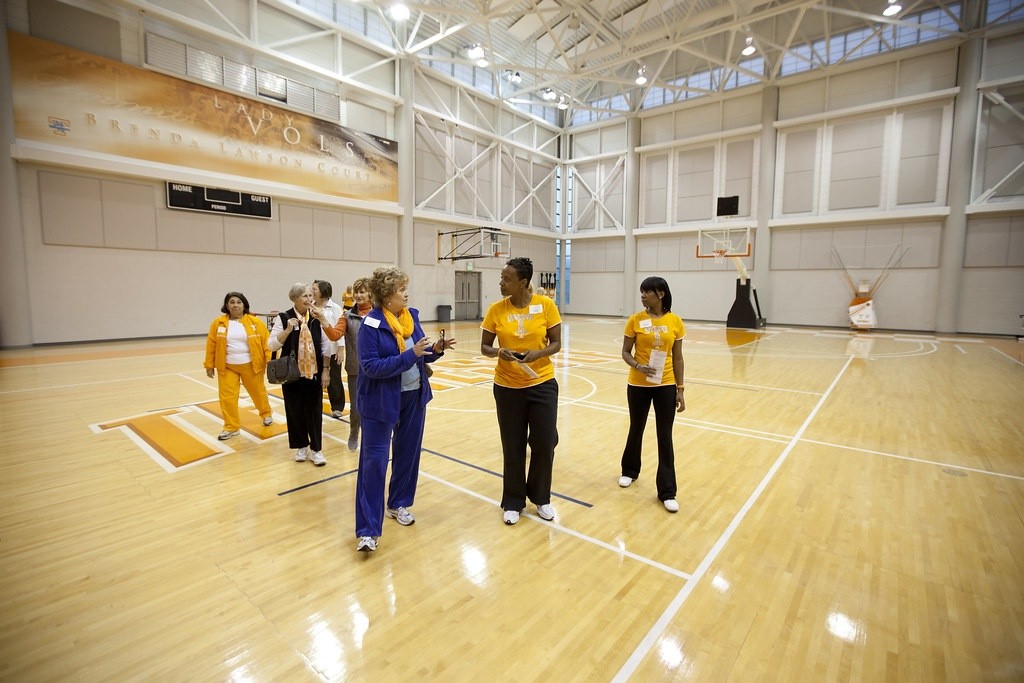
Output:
[267,311,300,383]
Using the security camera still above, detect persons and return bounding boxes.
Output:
[342,286,354,310]
[308,277,373,449]
[204,292,273,439]
[267,283,331,466]
[616,277,685,512]
[312,279,345,417]
[354,267,457,551]
[479,258,562,525]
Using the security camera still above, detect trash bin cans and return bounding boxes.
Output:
[437,305,452,322]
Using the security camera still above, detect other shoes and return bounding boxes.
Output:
[348,432,358,450]
[619,475,634,486]
[663,498,678,511]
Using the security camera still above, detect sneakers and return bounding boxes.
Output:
[218,429,239,440]
[386,506,414,526]
[356,535,378,551]
[308,450,325,465]
[332,410,340,417]
[503,509,519,523]
[295,447,308,461]
[536,503,554,520]
[263,415,272,425]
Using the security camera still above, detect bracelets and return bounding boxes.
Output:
[635,362,640,369]
[323,366,330,369]
[677,385,684,391]
[498,347,503,358]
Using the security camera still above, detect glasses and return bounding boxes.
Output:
[439,329,444,350]
